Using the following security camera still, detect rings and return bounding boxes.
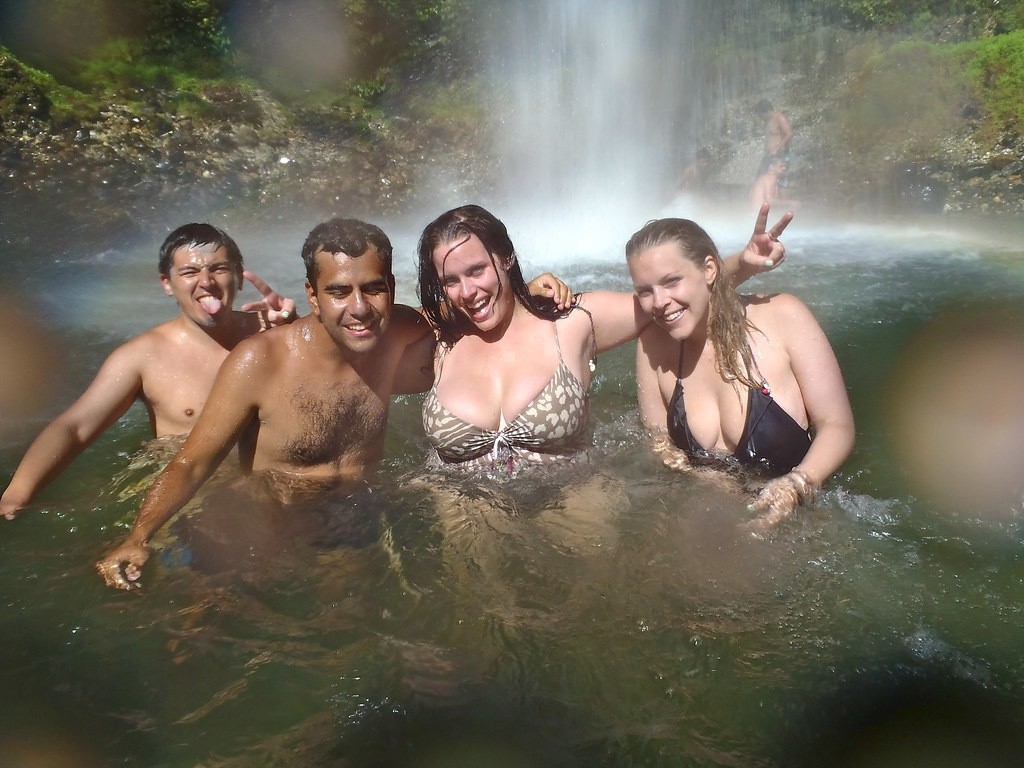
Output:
[768,232,777,241]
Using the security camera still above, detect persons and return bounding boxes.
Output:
[239,200,793,466]
[628,219,858,497]
[93,217,576,596]
[0,224,269,519]
